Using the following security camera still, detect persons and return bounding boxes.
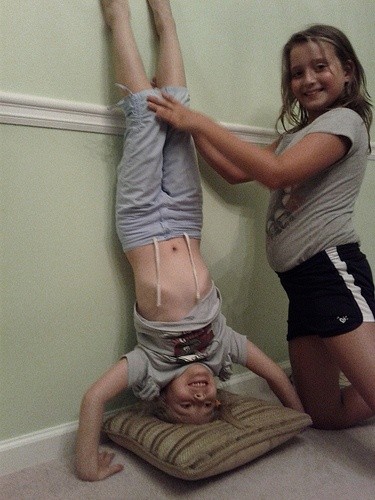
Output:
[145,23,375,430]
[73,1,305,483]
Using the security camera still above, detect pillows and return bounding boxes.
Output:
[102,388,313,482]
